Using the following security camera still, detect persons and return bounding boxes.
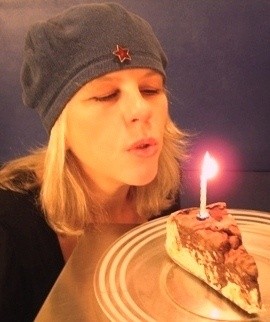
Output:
[0,3,202,322]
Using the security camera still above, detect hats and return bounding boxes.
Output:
[19,3,168,138]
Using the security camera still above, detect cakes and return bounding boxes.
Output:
[165,202,266,315]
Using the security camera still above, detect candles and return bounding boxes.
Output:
[198,150,210,219]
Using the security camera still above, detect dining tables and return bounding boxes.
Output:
[34,223,140,322]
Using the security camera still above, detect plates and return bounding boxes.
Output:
[93,209,270,322]
